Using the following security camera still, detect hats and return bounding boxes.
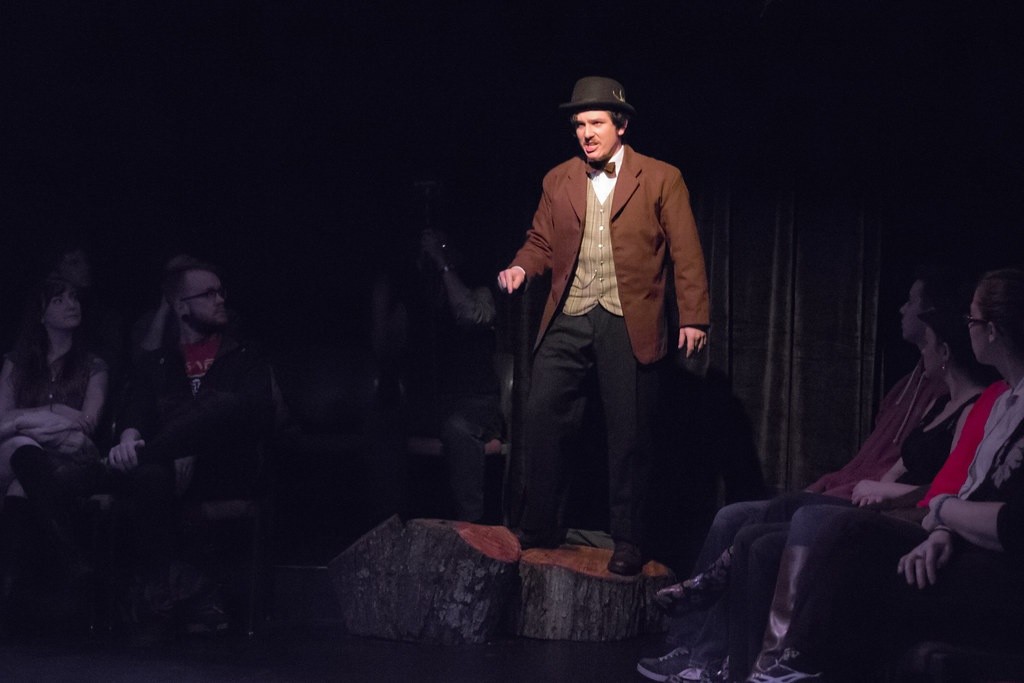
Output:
[559,77,635,117]
[917,309,971,352]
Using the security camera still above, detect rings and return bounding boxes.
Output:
[85,415,90,420]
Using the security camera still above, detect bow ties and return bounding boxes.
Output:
[584,160,616,175]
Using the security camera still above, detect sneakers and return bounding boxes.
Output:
[745,648,825,683]
[636,647,693,682]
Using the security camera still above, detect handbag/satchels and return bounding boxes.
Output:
[0,409,102,471]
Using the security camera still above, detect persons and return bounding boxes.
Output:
[496,75,711,576]
[1,163,504,640]
[634,267,1023,683]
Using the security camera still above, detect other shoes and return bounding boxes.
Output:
[100,444,155,481]
[648,568,721,612]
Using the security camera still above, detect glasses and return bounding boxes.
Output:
[962,312,988,328]
[178,285,230,302]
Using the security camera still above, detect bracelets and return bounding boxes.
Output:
[49,403,53,412]
[935,495,959,527]
[928,528,955,539]
[438,262,456,274]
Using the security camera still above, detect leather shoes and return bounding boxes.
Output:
[608,541,642,576]
[513,528,562,550]
